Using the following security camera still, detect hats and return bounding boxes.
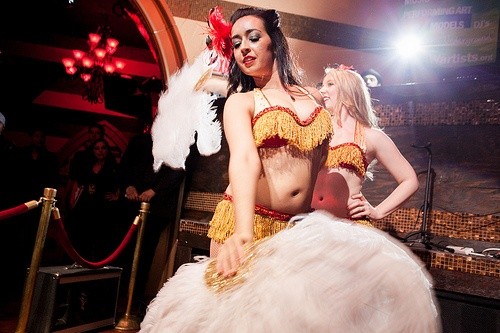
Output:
[361,68,383,83]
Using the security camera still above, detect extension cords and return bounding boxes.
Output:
[446,245,475,256]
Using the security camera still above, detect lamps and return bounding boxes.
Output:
[62,18,125,102]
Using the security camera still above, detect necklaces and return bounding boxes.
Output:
[263,83,291,99]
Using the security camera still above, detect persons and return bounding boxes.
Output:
[1,109,184,266]
[311,64,420,221]
[358,65,384,91]
[206,4,333,278]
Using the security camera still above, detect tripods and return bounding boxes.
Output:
[397,141,455,253]
